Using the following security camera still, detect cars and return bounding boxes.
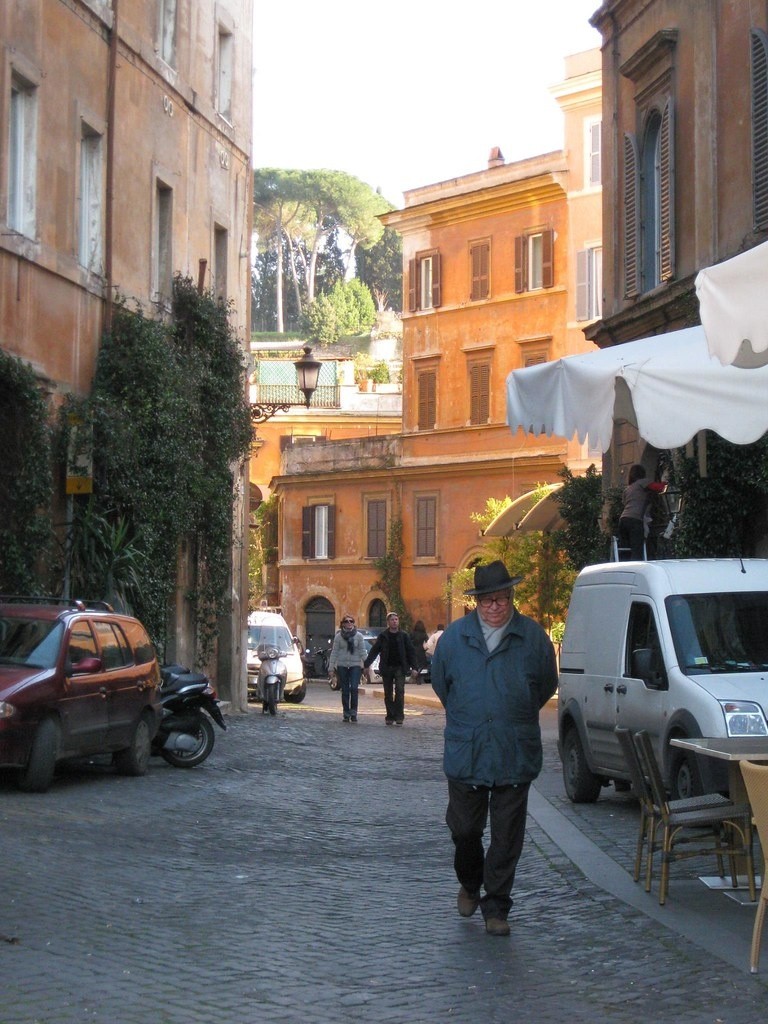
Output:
[247,610,308,703]
[324,625,415,688]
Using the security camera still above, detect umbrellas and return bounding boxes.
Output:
[694,237,768,369]
[506,322,768,452]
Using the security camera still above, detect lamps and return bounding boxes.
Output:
[249,346,321,425]
[658,480,684,525]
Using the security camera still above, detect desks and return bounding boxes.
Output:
[668,737,768,906]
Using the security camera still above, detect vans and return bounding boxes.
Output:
[554,559,768,802]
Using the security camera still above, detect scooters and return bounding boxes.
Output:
[248,645,289,716]
[158,665,230,767]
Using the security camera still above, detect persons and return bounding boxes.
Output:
[328,615,369,723]
[431,561,558,934]
[411,620,444,685]
[364,613,413,726]
[620,464,654,561]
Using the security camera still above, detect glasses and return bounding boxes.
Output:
[343,620,354,623]
[478,589,512,607]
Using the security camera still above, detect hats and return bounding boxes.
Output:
[464,560,521,596]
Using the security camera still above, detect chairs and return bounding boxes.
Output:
[738,759,768,973]
[614,724,757,906]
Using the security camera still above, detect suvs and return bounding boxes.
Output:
[0,595,159,792]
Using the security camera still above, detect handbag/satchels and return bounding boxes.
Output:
[328,667,341,691]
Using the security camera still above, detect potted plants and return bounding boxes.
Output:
[352,351,389,391]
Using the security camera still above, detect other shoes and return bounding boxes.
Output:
[343,716,349,722]
[386,720,394,725]
[396,719,402,724]
[351,715,357,722]
[485,914,510,937]
[457,882,481,916]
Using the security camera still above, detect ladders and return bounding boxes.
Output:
[609,535,648,562]
